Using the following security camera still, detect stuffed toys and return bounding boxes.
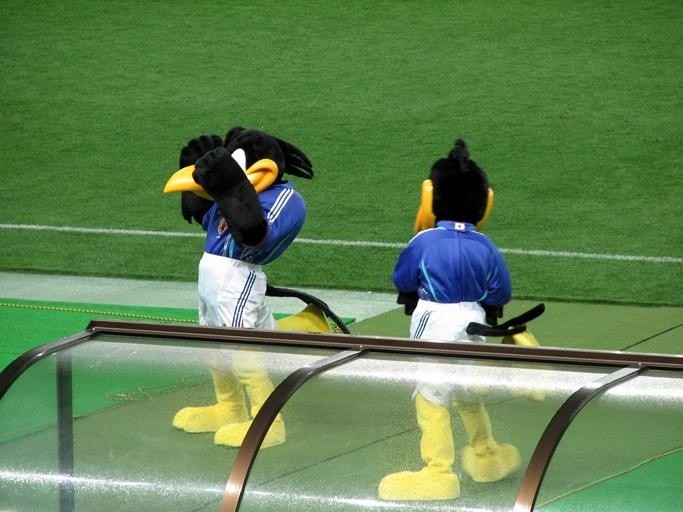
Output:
[375,136,556,503]
[163,125,351,451]
